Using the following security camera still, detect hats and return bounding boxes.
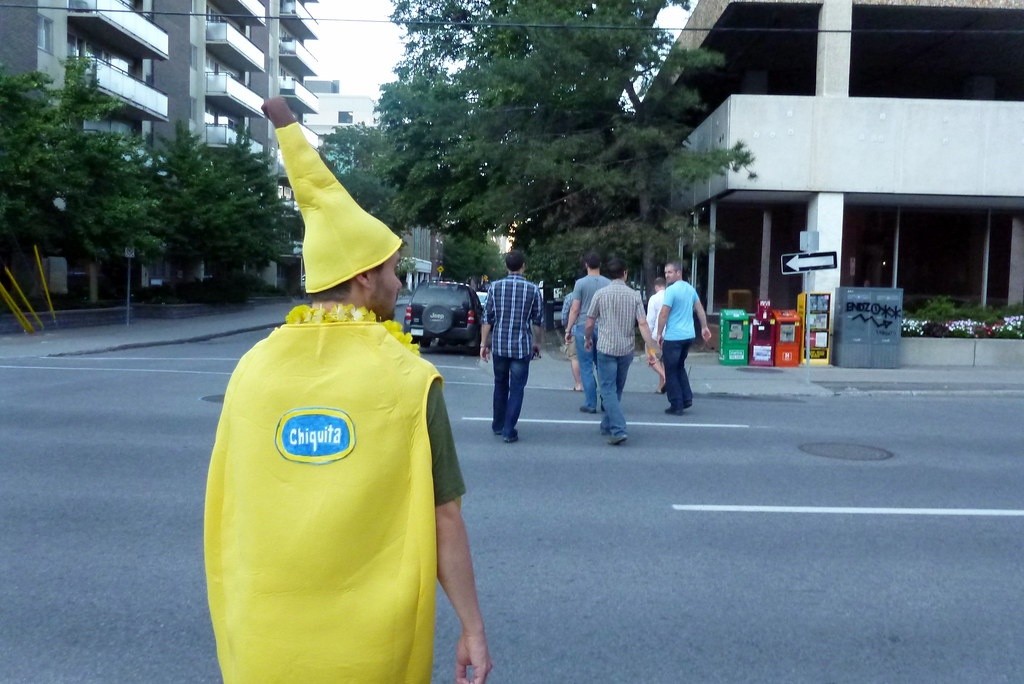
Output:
[262,95,402,293]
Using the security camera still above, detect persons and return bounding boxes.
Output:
[480,250,545,441]
[202,244,493,684]
[645,277,666,394]
[657,260,711,415]
[565,253,612,414]
[584,258,657,445]
[560,292,582,391]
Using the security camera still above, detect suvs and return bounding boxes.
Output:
[403,281,483,355]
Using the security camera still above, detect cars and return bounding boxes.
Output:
[476,292,488,309]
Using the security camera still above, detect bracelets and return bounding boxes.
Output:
[584,336,587,340]
[648,348,654,354]
[480,344,487,347]
[565,329,570,332]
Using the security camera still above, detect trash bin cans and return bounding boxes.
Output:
[719,308,749,368]
[772,308,801,368]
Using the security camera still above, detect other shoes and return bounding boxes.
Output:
[581,405,596,413]
[600,428,610,434]
[503,435,518,442]
[665,407,682,414]
[660,385,666,392]
[608,435,626,445]
[495,430,503,435]
[683,400,692,408]
